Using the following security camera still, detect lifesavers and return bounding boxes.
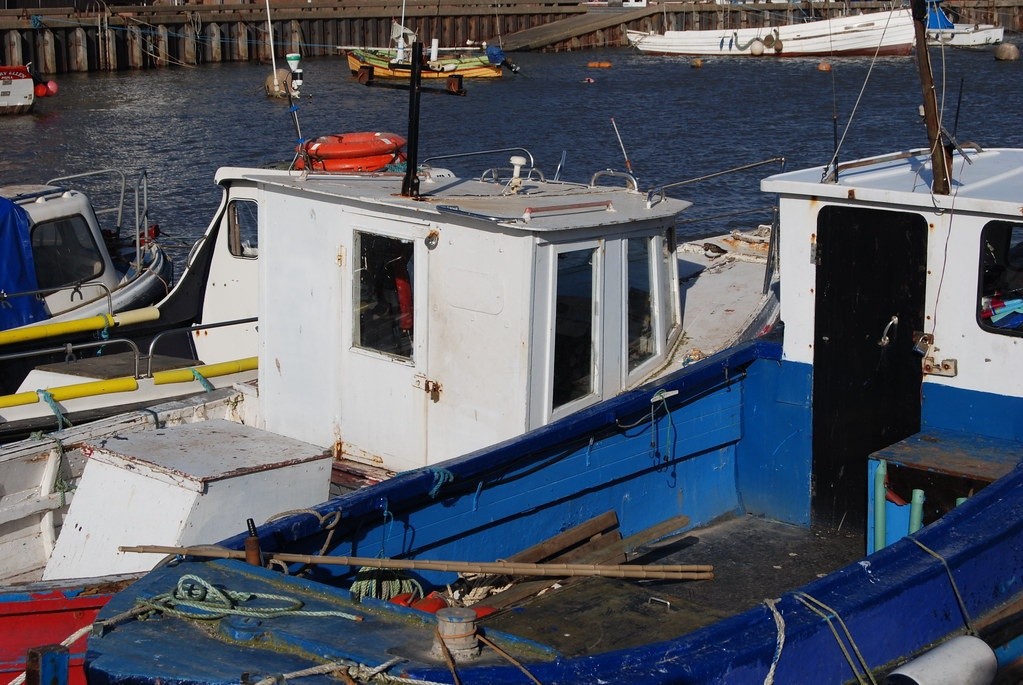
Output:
[291,131,409,173]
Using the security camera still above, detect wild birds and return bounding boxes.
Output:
[702,243,728,261]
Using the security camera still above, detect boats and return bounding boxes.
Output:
[625,0,928,60]
[0,46,786,685]
[0,61,34,114]
[920,23,1003,46]
[84,1,1023,684]
[0,56,458,440]
[0,169,172,334]
[347,0,502,94]
